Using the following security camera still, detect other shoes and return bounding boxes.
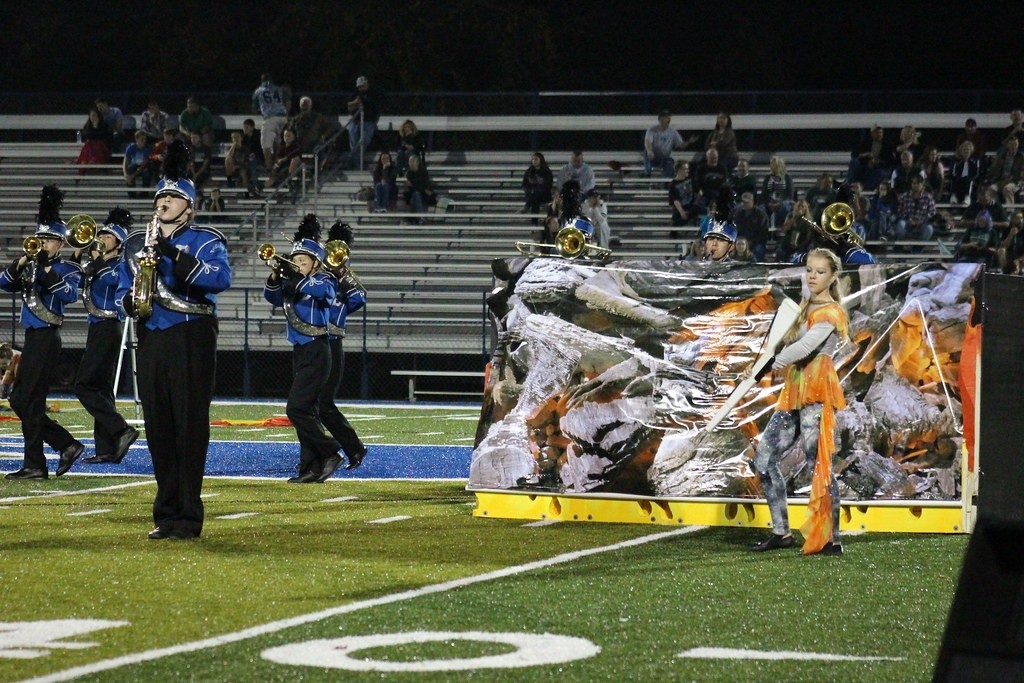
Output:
[751,533,792,551]
[818,541,843,557]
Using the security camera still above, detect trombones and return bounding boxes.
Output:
[515,227,612,258]
[64,213,109,258]
[799,201,864,252]
[279,230,369,298]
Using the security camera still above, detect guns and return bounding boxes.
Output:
[705,297,803,434]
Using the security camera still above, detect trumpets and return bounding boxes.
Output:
[23,237,41,254]
[258,243,302,276]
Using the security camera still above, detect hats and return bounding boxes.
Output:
[288,213,325,263]
[151,140,195,210]
[325,220,354,266]
[701,183,737,244]
[871,123,881,132]
[659,109,671,118]
[31,183,67,243]
[850,223,866,242]
[560,181,593,236]
[95,205,132,245]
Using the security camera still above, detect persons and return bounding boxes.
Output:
[265,214,368,483]
[516,151,611,258]
[374,120,436,225]
[742,249,848,553]
[640,110,935,265]
[0,184,140,482]
[78,73,380,223]
[917,108,1024,275]
[114,139,231,542]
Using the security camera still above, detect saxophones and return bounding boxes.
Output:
[133,205,167,321]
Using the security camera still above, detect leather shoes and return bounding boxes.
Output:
[287,444,368,483]
[82,448,121,465]
[168,528,198,542]
[113,426,140,463]
[4,461,49,479]
[55,440,86,477]
[146,525,169,540]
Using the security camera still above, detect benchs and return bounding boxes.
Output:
[0,140,1024,354]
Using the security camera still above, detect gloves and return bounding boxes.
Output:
[821,236,836,251]
[288,271,304,292]
[123,290,138,318]
[279,252,291,270]
[36,251,51,267]
[158,233,179,260]
[837,238,857,255]
